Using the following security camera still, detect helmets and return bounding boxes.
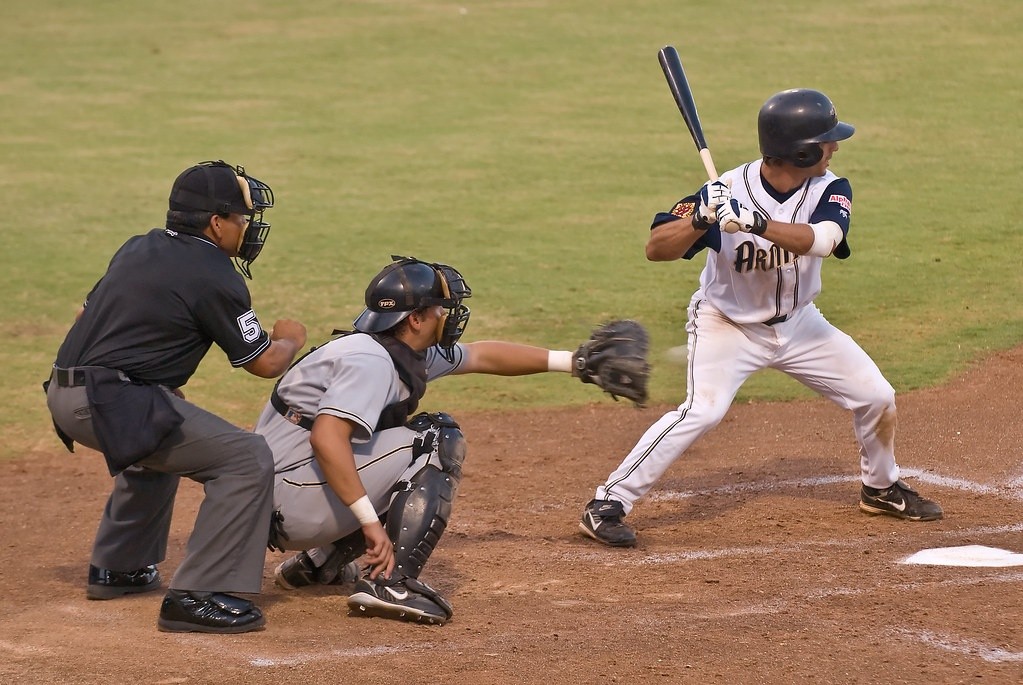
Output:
[353,258,439,333]
[758,88,857,170]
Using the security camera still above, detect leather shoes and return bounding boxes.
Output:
[86,561,161,600]
[159,587,266,634]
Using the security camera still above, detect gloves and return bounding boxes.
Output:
[691,176,732,231]
[715,196,768,236]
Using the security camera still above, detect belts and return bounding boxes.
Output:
[767,315,786,327]
[51,366,86,387]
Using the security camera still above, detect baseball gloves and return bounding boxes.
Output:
[571,318,651,406]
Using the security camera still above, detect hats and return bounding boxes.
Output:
[169,165,264,214]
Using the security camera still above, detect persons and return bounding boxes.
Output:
[254,254,651,627]
[44,160,310,634]
[576,89,942,549]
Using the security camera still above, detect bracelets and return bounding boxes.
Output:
[548,350,573,373]
[349,495,379,526]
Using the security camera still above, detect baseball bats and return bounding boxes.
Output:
[657,44,740,235]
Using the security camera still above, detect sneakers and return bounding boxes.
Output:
[274,551,361,589]
[579,496,636,547]
[860,482,944,522]
[345,574,448,626]
